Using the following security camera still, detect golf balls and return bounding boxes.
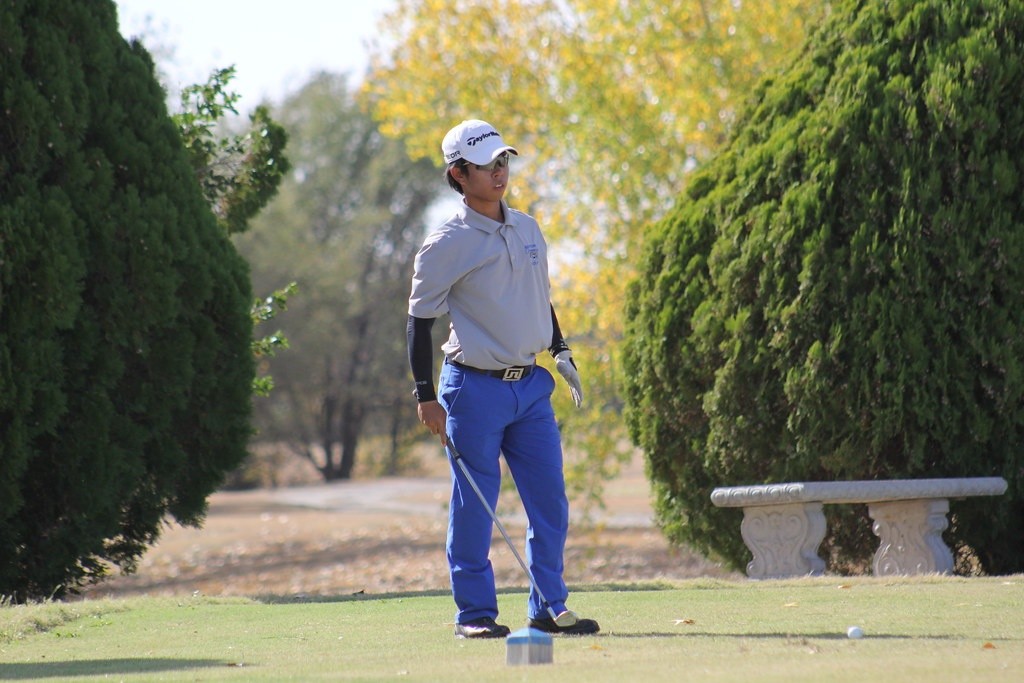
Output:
[848,626,862,639]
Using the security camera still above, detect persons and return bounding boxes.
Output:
[406,120,600,638]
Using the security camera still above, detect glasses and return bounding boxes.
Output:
[460,153,509,171]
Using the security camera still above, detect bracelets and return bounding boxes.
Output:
[549,343,567,356]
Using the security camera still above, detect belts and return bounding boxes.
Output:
[445,357,536,382]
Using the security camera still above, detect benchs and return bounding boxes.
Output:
[711,470,1010,580]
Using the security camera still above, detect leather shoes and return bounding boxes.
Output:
[455,616,510,639]
[529,611,600,636]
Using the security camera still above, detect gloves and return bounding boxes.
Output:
[554,350,582,408]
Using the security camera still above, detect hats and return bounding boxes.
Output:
[442,120,518,166]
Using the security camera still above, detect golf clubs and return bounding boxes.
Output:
[412,387,579,628]
